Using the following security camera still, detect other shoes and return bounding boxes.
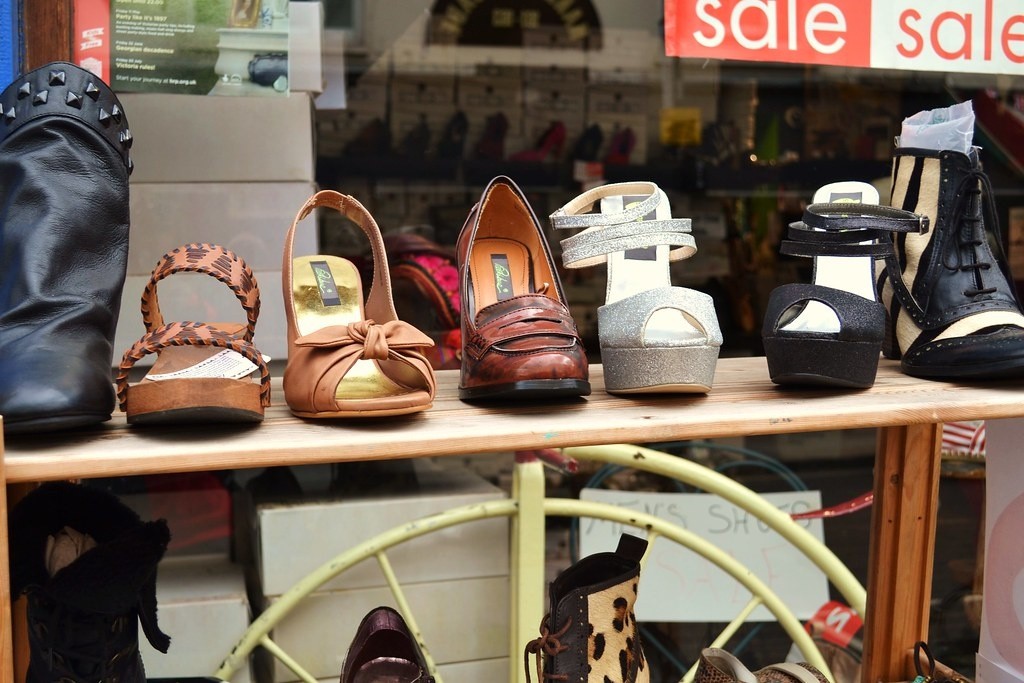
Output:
[340,605,435,683]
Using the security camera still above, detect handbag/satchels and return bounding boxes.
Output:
[693,647,831,683]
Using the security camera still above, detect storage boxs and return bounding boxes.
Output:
[135,552,256,683]
[265,573,510,683]
[240,459,510,598]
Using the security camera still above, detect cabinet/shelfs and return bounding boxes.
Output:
[1,177,1024,683]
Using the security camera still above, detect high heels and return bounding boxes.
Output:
[455,175,592,400]
[760,181,930,389]
[282,189,437,420]
[315,112,637,183]
[550,182,722,395]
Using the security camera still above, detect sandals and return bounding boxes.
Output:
[116,244,272,424]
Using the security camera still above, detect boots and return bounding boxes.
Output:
[524,533,650,683]
[9,481,171,683]
[0,62,133,429]
[875,146,1024,379]
